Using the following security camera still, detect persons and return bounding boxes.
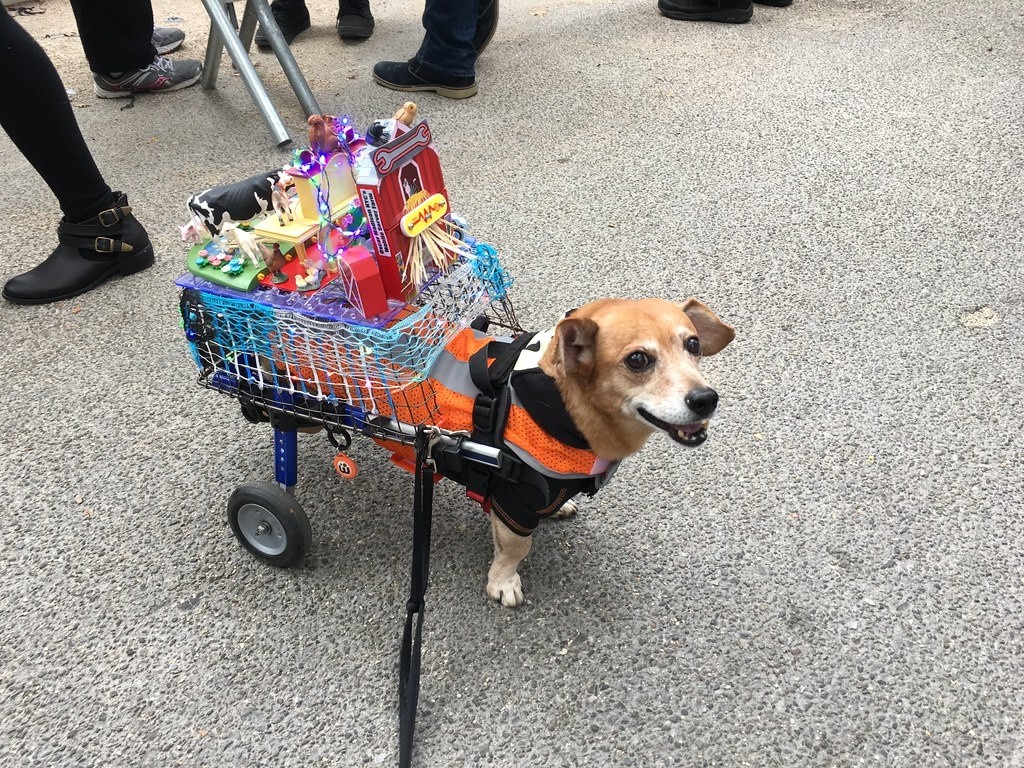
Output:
[70,0,204,99]
[256,0,374,45]
[658,0,793,23]
[0,0,155,305]
[371,0,499,99]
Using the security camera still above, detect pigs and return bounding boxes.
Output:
[177,216,209,246]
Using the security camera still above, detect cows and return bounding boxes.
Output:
[186,164,295,241]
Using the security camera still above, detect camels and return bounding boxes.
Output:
[307,114,343,153]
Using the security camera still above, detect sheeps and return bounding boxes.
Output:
[218,220,262,270]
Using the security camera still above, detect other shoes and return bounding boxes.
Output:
[658,0,753,23]
[472,0,500,59]
[754,0,793,6]
[372,58,478,98]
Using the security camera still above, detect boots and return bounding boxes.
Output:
[2,191,155,304]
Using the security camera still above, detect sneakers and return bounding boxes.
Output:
[151,27,185,54]
[94,56,201,98]
[255,0,311,44]
[336,0,375,38]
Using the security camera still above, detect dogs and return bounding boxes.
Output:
[261,292,727,605]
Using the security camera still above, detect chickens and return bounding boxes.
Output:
[254,241,286,279]
[291,259,324,287]
